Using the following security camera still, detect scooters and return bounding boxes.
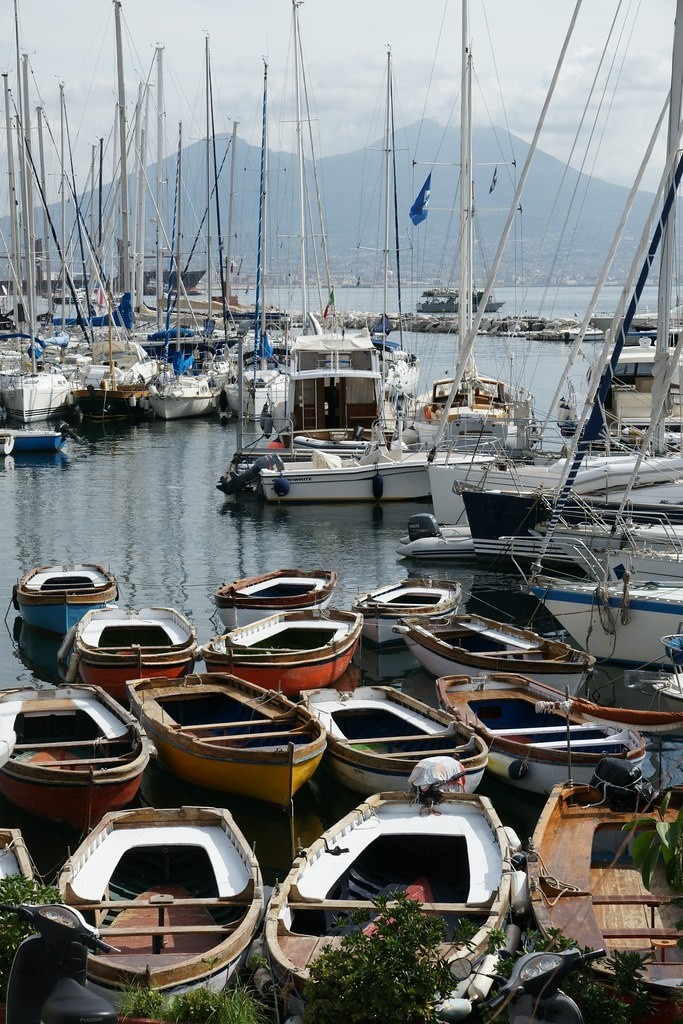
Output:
[447,948,606,1024]
[0,904,120,1024]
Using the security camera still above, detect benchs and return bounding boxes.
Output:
[12,586,683,953]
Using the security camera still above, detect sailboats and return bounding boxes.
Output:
[0,0,683,574]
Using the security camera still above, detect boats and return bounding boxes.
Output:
[262,755,512,1015]
[59,806,264,1013]
[528,489,683,582]
[435,672,646,796]
[75,606,197,711]
[16,563,118,635]
[214,569,338,632]
[415,289,509,314]
[527,757,683,1024]
[351,578,462,648]
[126,672,327,807]
[0,828,40,896]
[351,635,422,686]
[499,535,683,672]
[0,683,151,830]
[396,513,475,559]
[299,686,489,797]
[651,634,683,713]
[201,608,363,699]
[397,613,597,698]
[18,619,82,688]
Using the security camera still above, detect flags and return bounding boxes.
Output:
[409,173,431,226]
[489,167,497,194]
[324,291,334,318]
